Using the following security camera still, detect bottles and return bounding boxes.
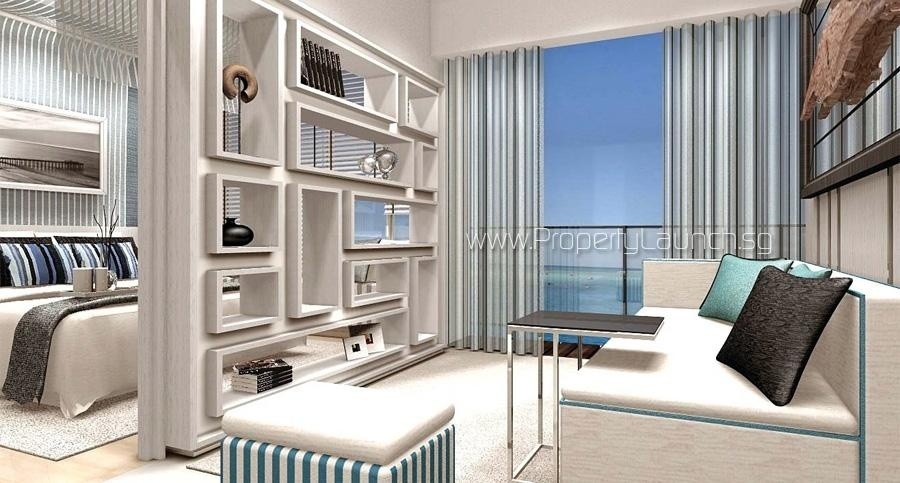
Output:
[222,218,254,247]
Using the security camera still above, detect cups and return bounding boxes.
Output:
[73,266,109,292]
